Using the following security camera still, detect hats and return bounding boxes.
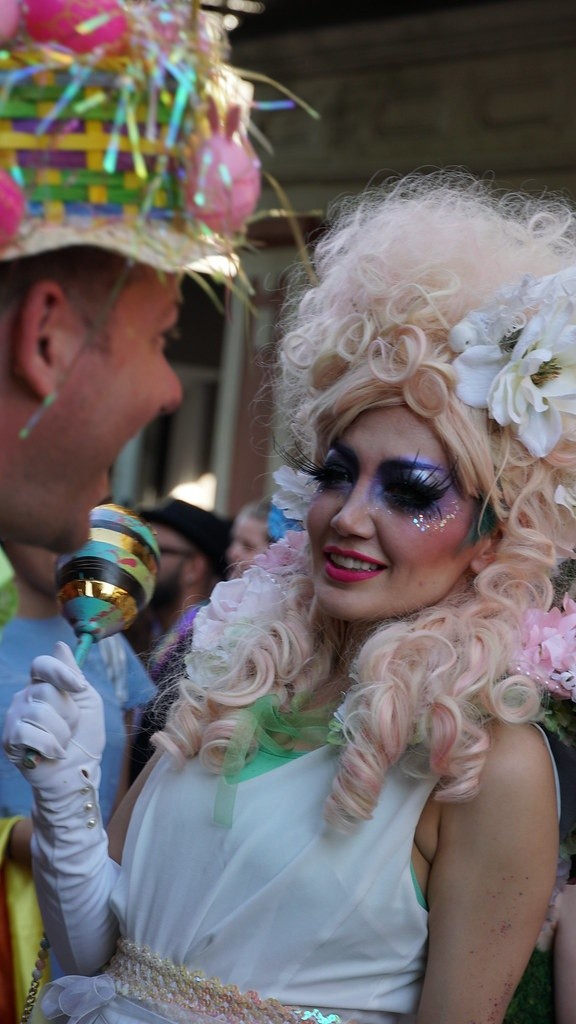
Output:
[138,499,234,582]
[0,0,324,316]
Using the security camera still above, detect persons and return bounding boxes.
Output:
[1,0,261,627]
[0,193,576,1024]
[1,466,158,867]
[129,498,275,785]
[504,884,575,1024]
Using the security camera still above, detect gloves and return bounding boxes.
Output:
[2,641,122,976]
